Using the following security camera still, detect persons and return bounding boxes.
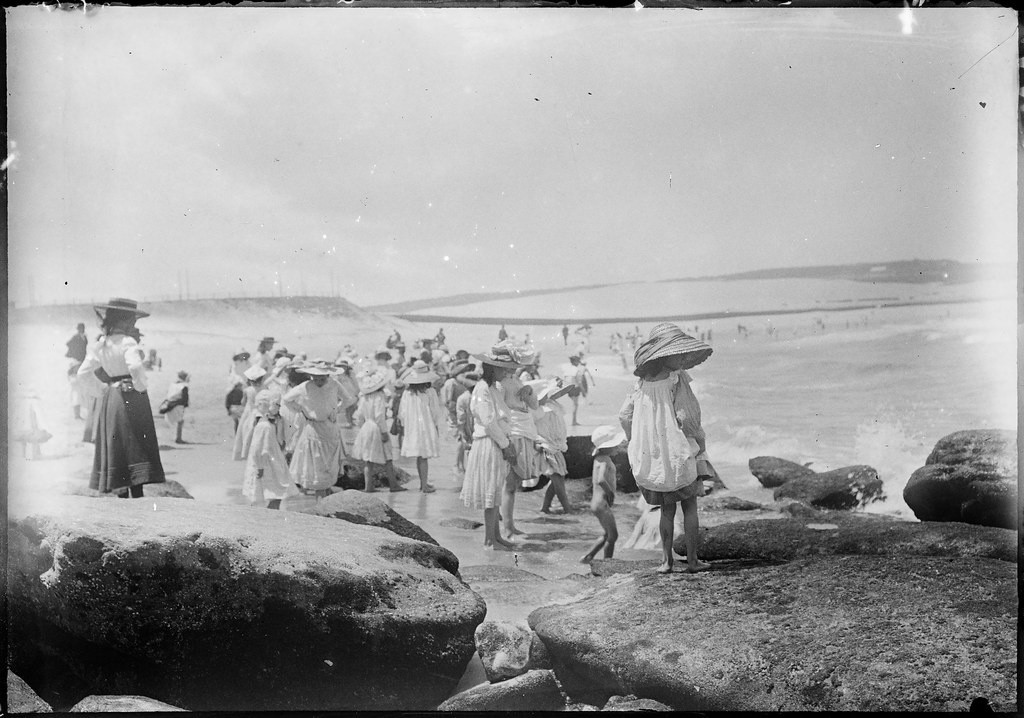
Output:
[618,323,713,573]
[457,346,548,551]
[562,324,569,345]
[533,376,576,515]
[280,363,354,499]
[241,389,299,510]
[397,359,443,493]
[609,332,637,351]
[624,495,682,552]
[221,325,541,460]
[695,325,712,341]
[579,425,626,564]
[138,347,162,371]
[351,375,408,492]
[65,324,88,420]
[563,353,595,425]
[159,369,191,444]
[77,296,167,499]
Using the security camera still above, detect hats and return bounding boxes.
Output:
[295,364,344,376]
[375,346,392,360]
[334,361,353,370]
[548,377,575,400]
[275,347,288,355]
[233,349,250,361]
[404,360,440,384]
[634,323,713,378]
[359,375,386,394]
[592,427,626,456]
[456,371,479,387]
[451,363,476,374]
[93,298,150,319]
[286,356,306,369]
[257,337,277,343]
[470,340,522,368]
